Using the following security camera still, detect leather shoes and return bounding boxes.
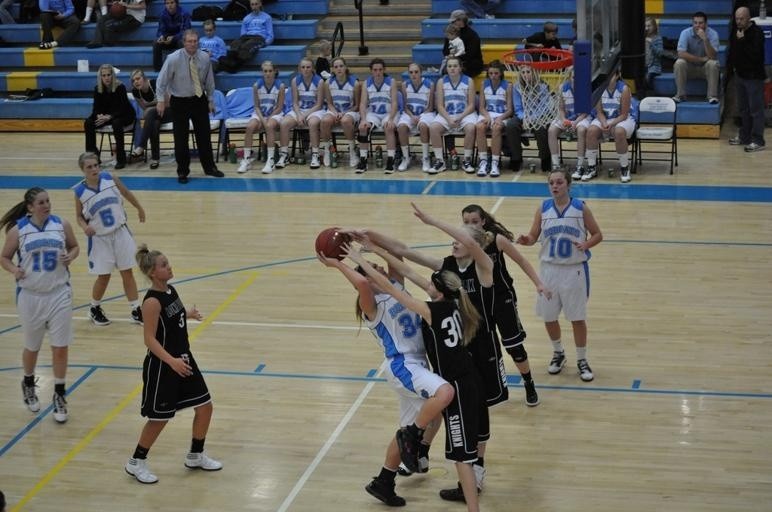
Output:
[204,166,225,178]
[176,173,190,184]
[128,149,146,159]
[148,160,161,170]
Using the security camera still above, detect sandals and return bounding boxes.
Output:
[37,41,54,52]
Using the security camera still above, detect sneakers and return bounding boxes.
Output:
[619,164,631,184]
[364,476,407,508]
[183,449,224,471]
[274,151,290,169]
[706,94,720,105]
[123,456,159,484]
[349,150,359,168]
[451,154,460,170]
[395,455,431,477]
[309,152,322,168]
[576,359,595,383]
[237,156,253,174]
[728,135,752,146]
[354,156,368,174]
[428,159,448,174]
[20,378,43,414]
[128,304,145,326]
[523,379,539,408]
[581,165,599,183]
[322,149,331,167]
[420,157,431,173]
[571,165,585,181]
[471,464,488,494]
[86,303,113,326]
[489,159,501,177]
[671,92,688,104]
[439,481,466,503]
[397,155,412,173]
[477,158,490,176]
[460,157,476,174]
[51,389,69,424]
[383,156,397,174]
[547,349,569,375]
[393,425,422,475]
[742,140,767,154]
[261,156,276,174]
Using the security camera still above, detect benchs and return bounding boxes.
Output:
[676,98,723,140]
[0,20,319,44]
[645,18,733,41]
[0,42,306,69]
[421,18,576,43]
[412,43,572,66]
[649,70,726,97]
[0,67,298,97]
[645,0,735,15]
[0,95,94,119]
[141,0,328,20]
[431,1,577,17]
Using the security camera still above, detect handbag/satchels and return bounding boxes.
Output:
[190,2,225,23]
[221,1,255,23]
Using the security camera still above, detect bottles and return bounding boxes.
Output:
[451,153,457,170]
[259,139,266,161]
[330,146,337,168]
[229,143,237,164]
[375,145,383,168]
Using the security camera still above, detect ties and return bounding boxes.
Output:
[188,55,204,99]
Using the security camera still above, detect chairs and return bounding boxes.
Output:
[522,131,542,167]
[188,89,225,162]
[330,125,360,167]
[368,90,404,164]
[284,86,311,164]
[224,87,265,161]
[594,96,635,172]
[95,94,138,162]
[635,97,678,175]
[434,92,480,167]
[144,121,178,163]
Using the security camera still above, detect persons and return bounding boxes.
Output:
[315,201,509,512]
[124,242,223,484]
[461,205,554,407]
[0,1,771,183]
[72,152,146,327]
[517,169,604,382]
[0,188,79,423]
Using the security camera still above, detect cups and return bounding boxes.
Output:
[529,163,536,173]
[608,168,614,177]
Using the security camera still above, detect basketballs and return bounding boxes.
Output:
[314,226,352,261]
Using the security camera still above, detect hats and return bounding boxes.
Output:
[447,8,467,24]
